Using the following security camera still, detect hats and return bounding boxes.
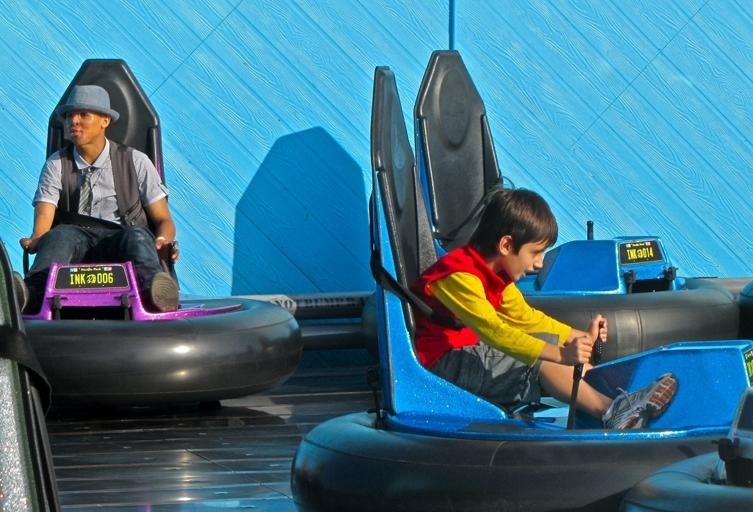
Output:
[55,85,120,124]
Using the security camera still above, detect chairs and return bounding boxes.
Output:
[365,65,539,421]
[413,48,505,254]
[45,58,168,205]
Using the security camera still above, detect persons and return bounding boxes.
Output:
[410,188,679,430]
[12,85,181,313]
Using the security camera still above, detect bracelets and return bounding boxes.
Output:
[155,236,166,242]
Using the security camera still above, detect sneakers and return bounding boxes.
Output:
[12,270,31,313]
[601,372,678,432]
[151,273,179,311]
